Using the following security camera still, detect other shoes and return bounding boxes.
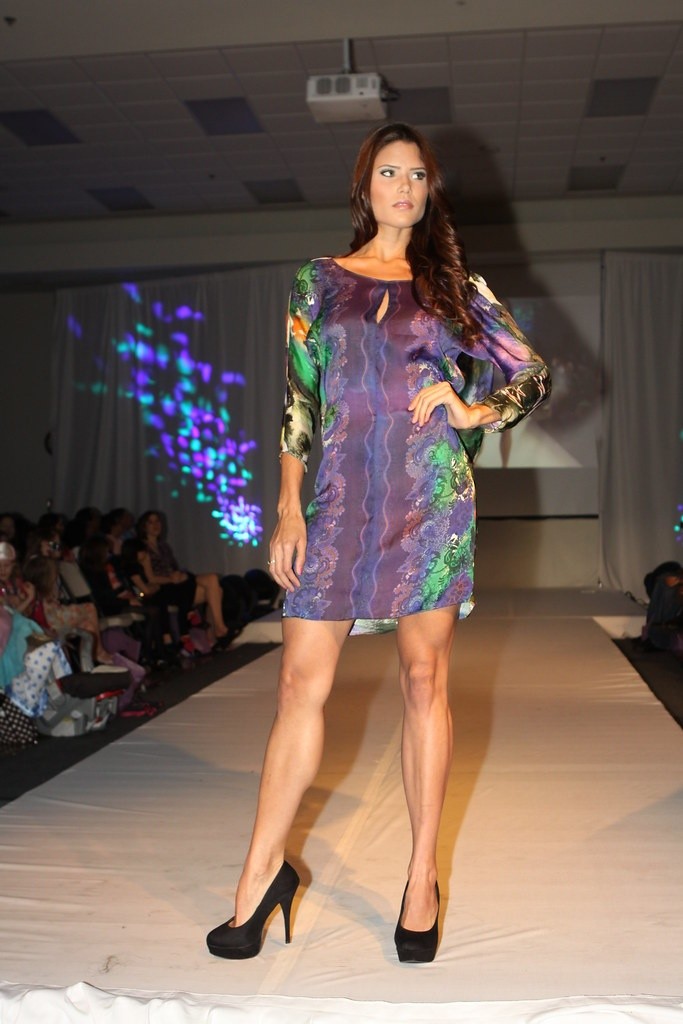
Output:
[120,701,160,717]
[217,627,240,650]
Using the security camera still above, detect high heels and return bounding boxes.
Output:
[394,869,441,965]
[205,859,300,958]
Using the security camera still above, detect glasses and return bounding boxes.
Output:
[43,540,61,551]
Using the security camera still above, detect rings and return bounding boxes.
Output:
[267,560,275,564]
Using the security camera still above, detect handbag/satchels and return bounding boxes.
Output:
[0,693,38,753]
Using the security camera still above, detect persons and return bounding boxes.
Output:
[0,506,243,758]
[206,119,551,962]
[641,561,683,671]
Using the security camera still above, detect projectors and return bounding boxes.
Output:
[304,72,388,122]
